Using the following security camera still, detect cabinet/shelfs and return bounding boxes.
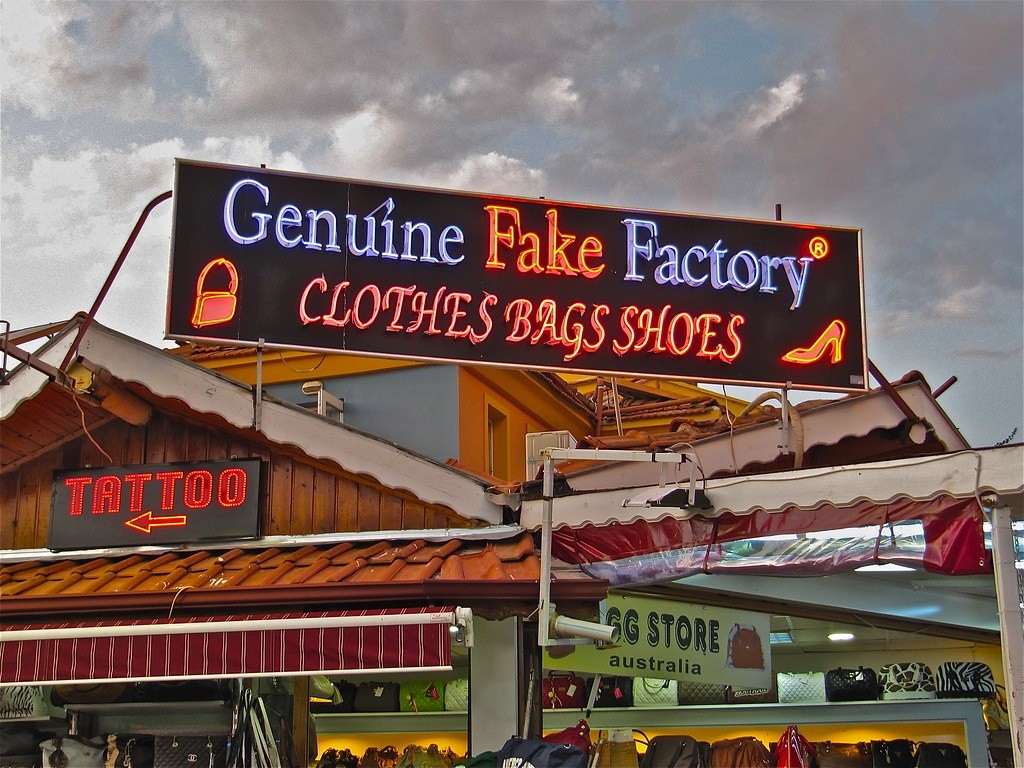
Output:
[311,698,989,768]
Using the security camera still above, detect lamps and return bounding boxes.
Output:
[622,452,712,510]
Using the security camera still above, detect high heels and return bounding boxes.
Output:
[987,700,1008,719]
[983,704,1009,730]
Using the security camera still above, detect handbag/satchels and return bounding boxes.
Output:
[354,681,400,711]
[773,725,815,768]
[284,675,342,705]
[401,680,445,711]
[912,742,968,768]
[709,736,773,768]
[315,719,639,768]
[0,677,282,768]
[586,676,633,706]
[542,670,585,708]
[812,741,872,768]
[678,683,726,703]
[776,672,826,702]
[879,663,936,699]
[640,734,710,768]
[634,677,678,706]
[310,681,353,713]
[870,739,913,768]
[728,673,778,702]
[446,679,468,711]
[826,666,879,700]
[936,662,997,700]
